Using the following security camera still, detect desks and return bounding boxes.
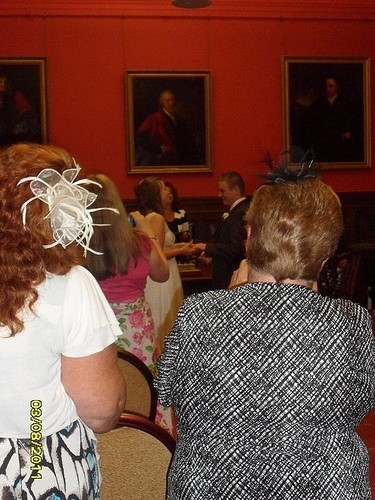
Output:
[181,267,212,299]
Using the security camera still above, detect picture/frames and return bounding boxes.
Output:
[281,54,372,171]
[124,69,214,175]
[0,56,50,150]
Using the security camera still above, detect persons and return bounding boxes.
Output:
[129,177,199,358]
[158,183,193,265]
[192,170,252,289]
[79,174,173,434]
[154,174,375,500]
[0,143,128,500]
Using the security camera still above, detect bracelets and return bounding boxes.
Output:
[149,236,160,242]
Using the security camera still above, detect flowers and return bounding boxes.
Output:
[222,212,229,221]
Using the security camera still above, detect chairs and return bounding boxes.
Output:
[117,349,158,423]
[94,411,175,500]
